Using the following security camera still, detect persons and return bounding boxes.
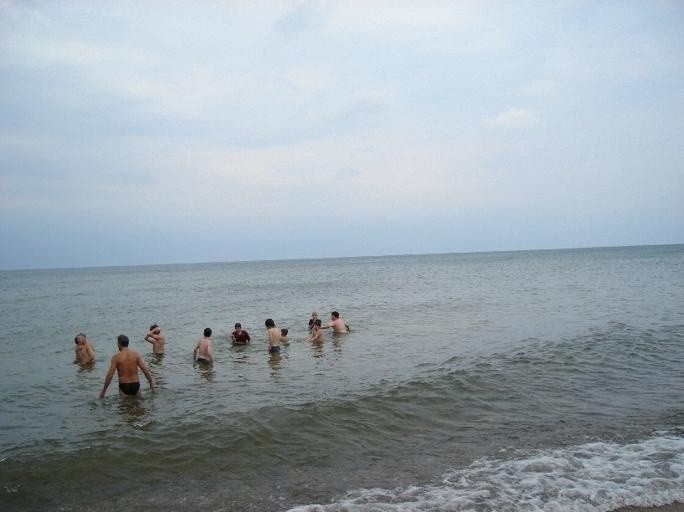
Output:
[279,328,289,346]
[265,319,281,354]
[309,312,318,334]
[137,324,164,370]
[193,328,213,370]
[98,335,156,400]
[309,319,323,344]
[75,333,96,365]
[319,310,349,334]
[231,323,250,345]
